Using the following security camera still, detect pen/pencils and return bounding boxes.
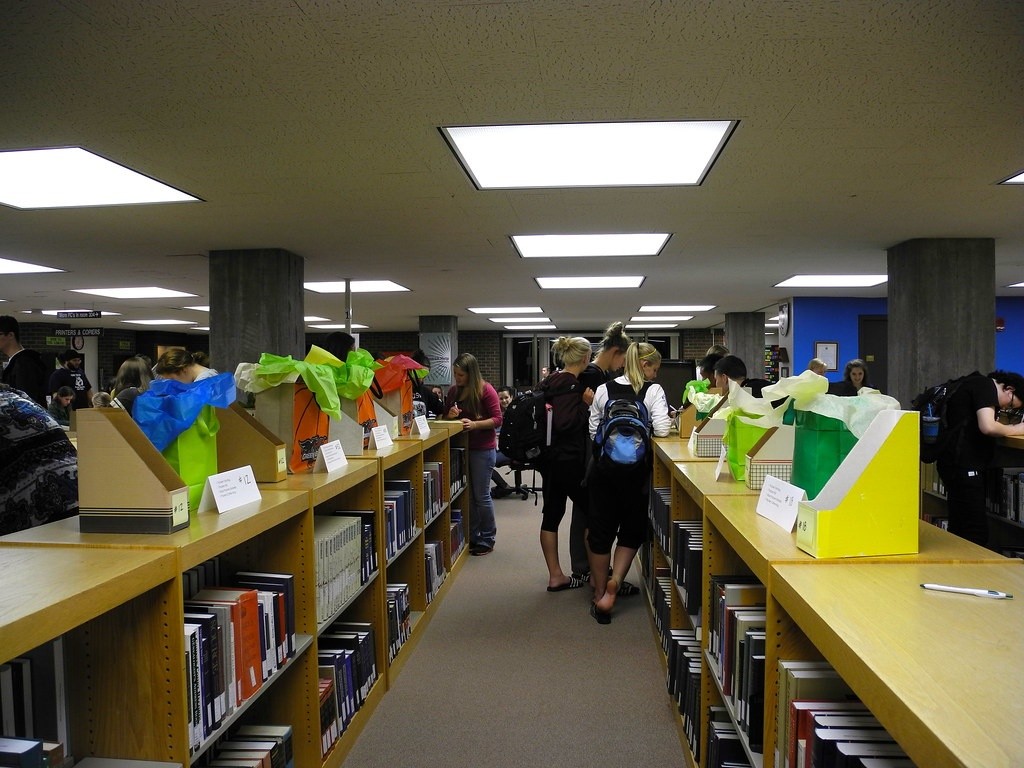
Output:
[1021,414,1024,422]
[920,584,1013,599]
[669,405,677,411]
[455,402,457,408]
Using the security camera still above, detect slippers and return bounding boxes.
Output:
[590,602,612,625]
[572,571,592,582]
[617,582,639,595]
[548,576,583,591]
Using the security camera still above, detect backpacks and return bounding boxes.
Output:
[498,376,577,463]
[912,371,978,464]
[592,380,653,480]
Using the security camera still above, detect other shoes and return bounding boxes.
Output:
[489,486,511,498]
[469,542,493,556]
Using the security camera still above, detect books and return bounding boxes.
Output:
[424,446,469,604]
[316,516,379,755]
[182,558,299,767]
[640,485,703,763]
[0,637,77,767]
[920,462,1024,561]
[384,479,419,665]
[707,577,919,768]
[764,345,779,384]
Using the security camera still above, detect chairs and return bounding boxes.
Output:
[493,462,543,501]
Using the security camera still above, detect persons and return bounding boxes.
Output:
[321,331,356,363]
[937,368,1024,537]
[541,321,679,624]
[701,342,775,398]
[1,316,95,536]
[809,357,866,395]
[412,348,447,419]
[92,348,219,418]
[447,354,514,555]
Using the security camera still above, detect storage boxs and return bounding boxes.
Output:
[678,388,921,559]
[75,369,413,535]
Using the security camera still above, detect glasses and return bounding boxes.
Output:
[1006,391,1014,409]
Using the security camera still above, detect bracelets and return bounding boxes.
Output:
[473,422,477,429]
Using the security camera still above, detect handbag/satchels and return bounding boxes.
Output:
[407,367,444,413]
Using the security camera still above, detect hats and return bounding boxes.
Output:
[63,350,80,361]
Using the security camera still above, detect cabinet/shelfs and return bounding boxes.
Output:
[0,421,468,768]
[634,428,1023,768]
[922,435,1024,547]
